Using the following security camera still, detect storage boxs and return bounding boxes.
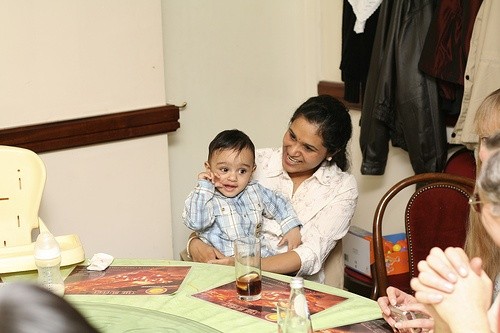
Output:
[342,227,376,278]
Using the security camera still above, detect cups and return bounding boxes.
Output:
[234,237,263,302]
[276,300,290,333]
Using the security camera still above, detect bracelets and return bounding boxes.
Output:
[187,235,198,256]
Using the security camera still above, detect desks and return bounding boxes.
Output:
[0,259,395,333]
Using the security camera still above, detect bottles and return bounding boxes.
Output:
[33,233,65,299]
[288,277,312,333]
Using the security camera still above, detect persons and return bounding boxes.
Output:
[184,130,302,259]
[410,131,500,333]
[0,283,103,333]
[186,94,358,289]
[378,89,500,333]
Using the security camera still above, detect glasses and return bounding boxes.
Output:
[469,193,500,213]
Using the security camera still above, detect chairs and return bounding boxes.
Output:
[323,148,481,301]
[0,146,85,274]
